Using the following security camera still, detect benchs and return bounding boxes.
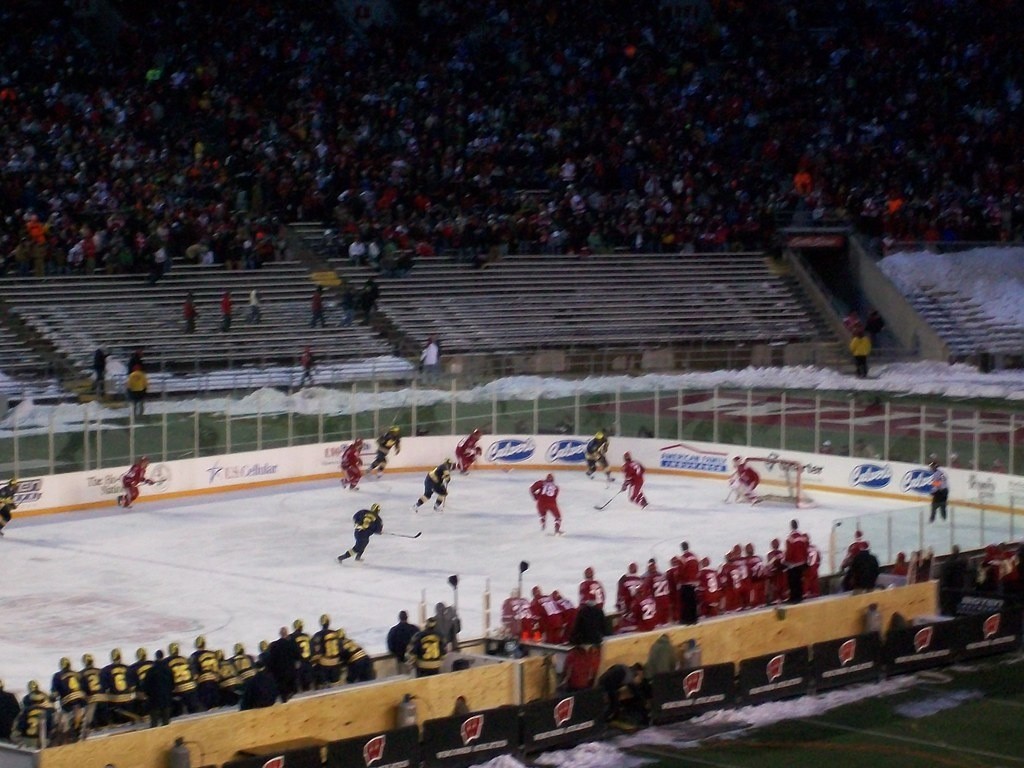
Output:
[0,222,1024,403]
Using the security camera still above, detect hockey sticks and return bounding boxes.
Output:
[482,454,515,475]
[381,531,423,540]
[388,394,410,428]
[16,482,38,507]
[442,481,450,510]
[138,477,168,487]
[594,489,623,510]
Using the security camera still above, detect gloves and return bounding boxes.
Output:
[621,485,627,492]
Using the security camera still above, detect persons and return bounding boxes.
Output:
[728,457,761,506]
[977,344,992,374]
[249,286,261,327]
[88,342,147,421]
[456,429,483,475]
[621,451,648,510]
[412,457,458,513]
[299,345,314,388]
[420,337,439,387]
[530,474,564,534]
[841,308,885,380]
[502,519,1024,735]
[365,425,401,478]
[585,432,616,482]
[220,291,232,333]
[341,437,363,490]
[0,602,460,748]
[0,479,23,536]
[309,289,327,329]
[929,461,948,522]
[183,292,200,334]
[118,456,155,509]
[338,503,383,564]
[0,0,1024,279]
[336,280,380,329]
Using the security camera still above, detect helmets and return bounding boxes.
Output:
[473,429,481,436]
[371,503,380,513]
[355,438,362,445]
[390,425,400,432]
[595,432,604,440]
[547,474,552,480]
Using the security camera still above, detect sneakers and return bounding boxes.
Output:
[413,503,418,513]
[434,506,442,511]
[341,479,347,488]
